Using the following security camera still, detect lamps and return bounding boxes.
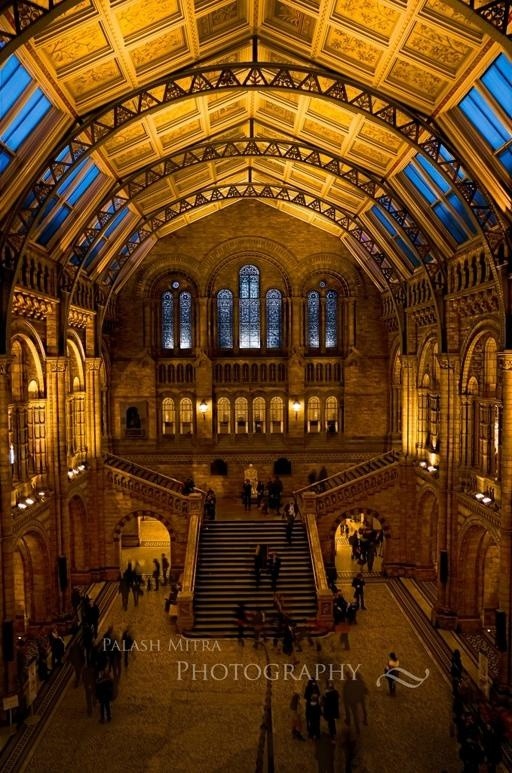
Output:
[292,397,301,420]
[199,396,208,420]
[471,491,492,506]
